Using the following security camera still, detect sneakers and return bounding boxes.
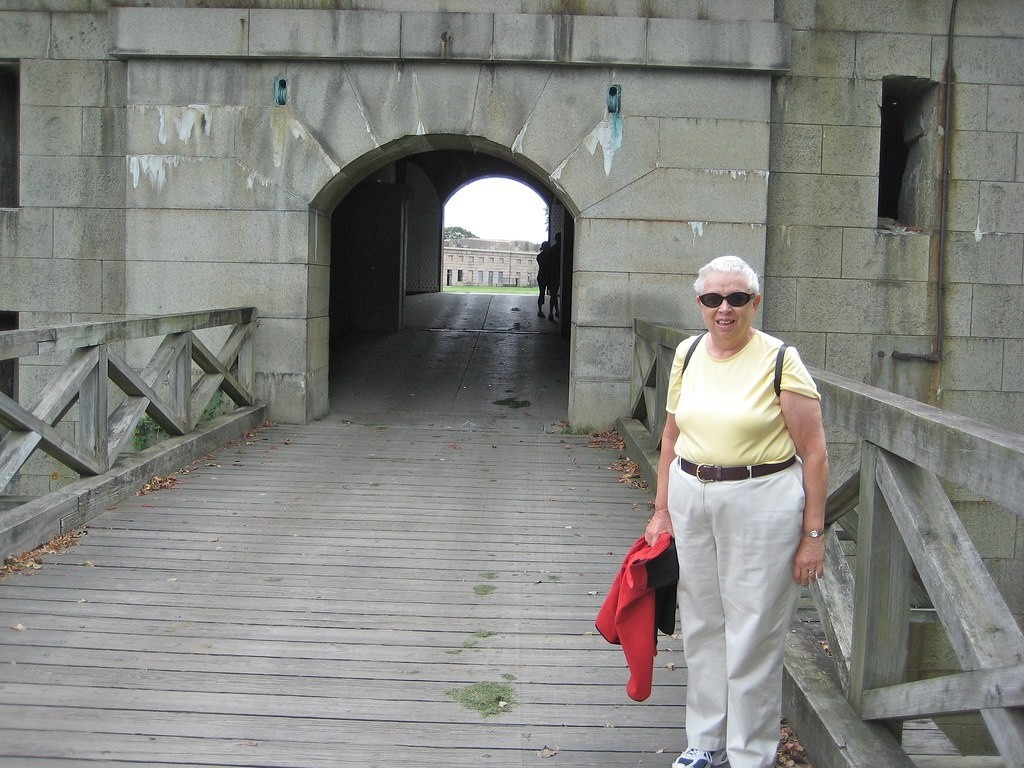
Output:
[672,745,727,768]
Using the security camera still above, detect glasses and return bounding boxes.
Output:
[698,292,755,308]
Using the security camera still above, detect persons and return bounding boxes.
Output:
[644,255,830,768]
[536,233,561,321]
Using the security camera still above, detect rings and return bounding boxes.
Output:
[809,569,814,573]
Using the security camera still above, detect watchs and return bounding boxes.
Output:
[803,529,825,539]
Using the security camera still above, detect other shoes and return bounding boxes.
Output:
[538,311,545,317]
[555,311,560,317]
[549,315,554,322]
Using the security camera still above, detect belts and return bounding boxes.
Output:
[678,455,797,484]
